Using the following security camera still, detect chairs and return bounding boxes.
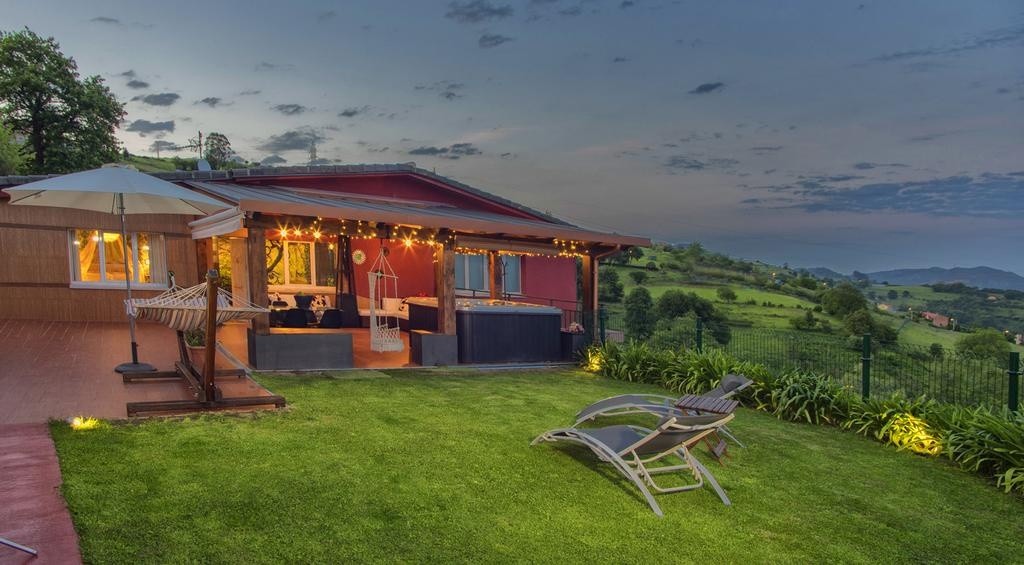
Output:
[525,376,753,516]
[268,293,362,328]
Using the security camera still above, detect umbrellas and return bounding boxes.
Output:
[2,162,236,365]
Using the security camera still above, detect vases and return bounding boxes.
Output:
[561,331,591,362]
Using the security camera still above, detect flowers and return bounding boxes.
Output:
[563,322,585,332]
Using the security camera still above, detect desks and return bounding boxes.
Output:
[661,409,735,467]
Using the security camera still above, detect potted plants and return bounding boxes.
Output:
[182,328,207,366]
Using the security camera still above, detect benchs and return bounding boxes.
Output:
[268,295,331,311]
[357,298,410,330]
